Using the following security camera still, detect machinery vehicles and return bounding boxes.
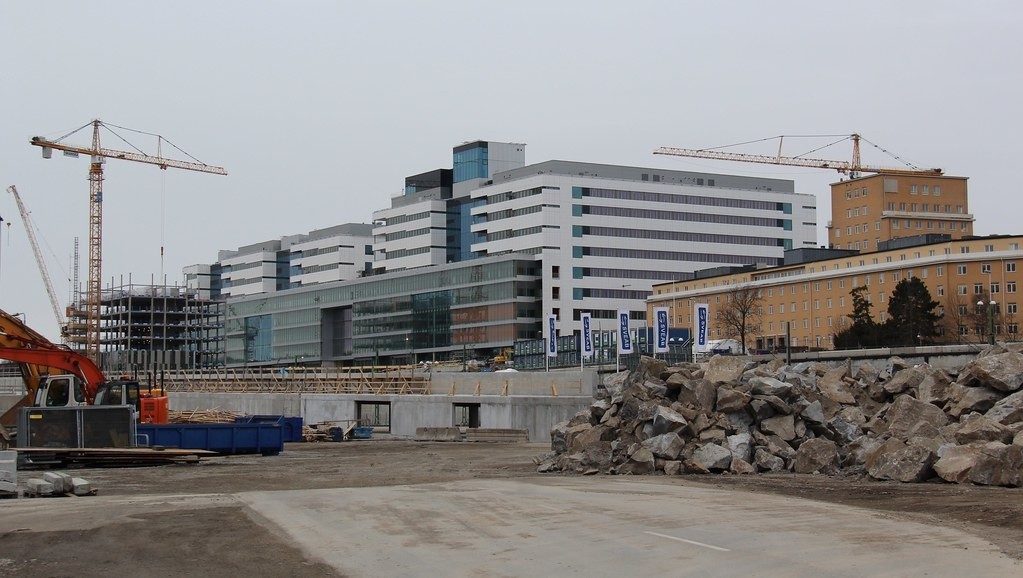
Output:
[493,350,514,364]
[0,309,170,449]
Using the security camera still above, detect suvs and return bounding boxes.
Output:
[749,348,770,355]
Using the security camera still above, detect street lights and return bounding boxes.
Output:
[671,272,675,328]
[917,333,921,347]
[295,355,304,368]
[689,297,696,340]
[977,300,997,343]
[985,270,992,304]
[711,331,715,356]
[406,338,414,365]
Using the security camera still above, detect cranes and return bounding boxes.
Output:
[653,133,945,179]
[6,185,78,351]
[27,120,229,369]
